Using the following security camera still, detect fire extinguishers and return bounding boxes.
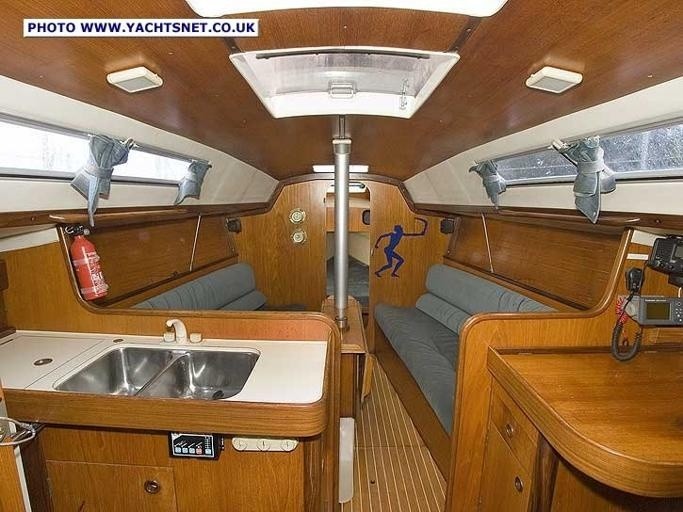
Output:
[63,222,109,303]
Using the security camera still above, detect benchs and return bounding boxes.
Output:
[374,255,583,484]
[99,254,305,312]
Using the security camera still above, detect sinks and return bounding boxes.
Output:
[56,346,171,396]
[139,349,261,398]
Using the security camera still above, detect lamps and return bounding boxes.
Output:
[526,64,584,95]
[106,66,164,95]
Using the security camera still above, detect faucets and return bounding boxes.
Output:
[166,319,187,339]
